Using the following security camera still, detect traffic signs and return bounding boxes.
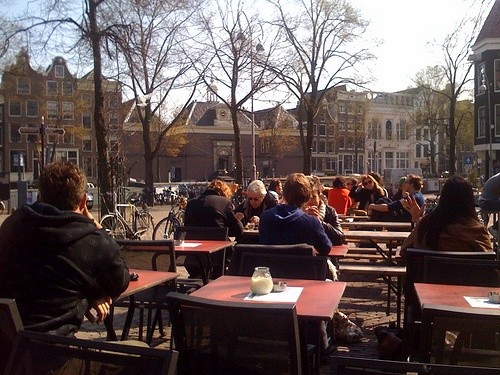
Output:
[18,127,39,134]
[46,128,65,135]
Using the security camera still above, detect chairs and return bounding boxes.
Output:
[397,247,498,301]
[110,239,191,341]
[164,292,317,375]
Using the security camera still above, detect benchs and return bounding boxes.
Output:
[1,330,179,374]
[330,348,499,375]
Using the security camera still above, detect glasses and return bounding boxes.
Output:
[248,197,262,201]
[363,180,372,186]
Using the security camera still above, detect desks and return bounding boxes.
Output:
[179,276,347,367]
[104,202,500,286]
[412,282,500,350]
[82,267,181,342]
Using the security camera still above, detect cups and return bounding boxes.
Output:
[247,222,255,232]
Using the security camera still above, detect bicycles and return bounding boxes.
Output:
[99,192,154,240]
[151,198,187,241]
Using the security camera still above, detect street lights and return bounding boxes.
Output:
[236,11,265,180]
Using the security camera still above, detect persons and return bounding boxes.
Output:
[256,173,338,359]
[152,183,204,206]
[348,174,387,263]
[358,174,426,255]
[234,180,278,229]
[327,177,353,216]
[398,177,493,259]
[0,160,152,375]
[478,171,500,239]
[183,180,245,281]
[301,175,346,246]
[268,179,286,205]
[346,177,407,202]
